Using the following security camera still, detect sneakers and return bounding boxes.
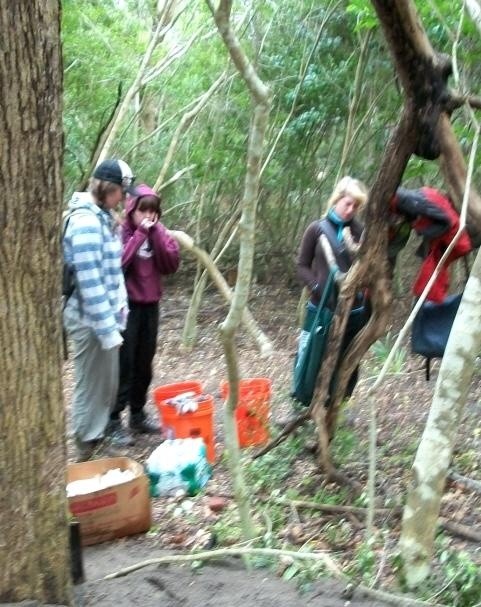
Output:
[130,410,161,431]
[103,420,135,447]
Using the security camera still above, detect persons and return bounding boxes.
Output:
[273,176,370,432]
[103,183,181,449]
[59,157,139,463]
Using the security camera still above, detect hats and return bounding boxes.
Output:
[94,159,139,197]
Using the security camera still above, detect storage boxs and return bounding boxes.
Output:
[66,456,150,547]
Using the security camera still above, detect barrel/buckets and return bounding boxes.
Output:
[158,396,216,464]
[221,379,273,449]
[153,383,202,408]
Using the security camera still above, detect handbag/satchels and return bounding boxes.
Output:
[293,264,365,407]
[413,292,463,357]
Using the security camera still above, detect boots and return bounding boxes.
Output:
[75,440,102,461]
